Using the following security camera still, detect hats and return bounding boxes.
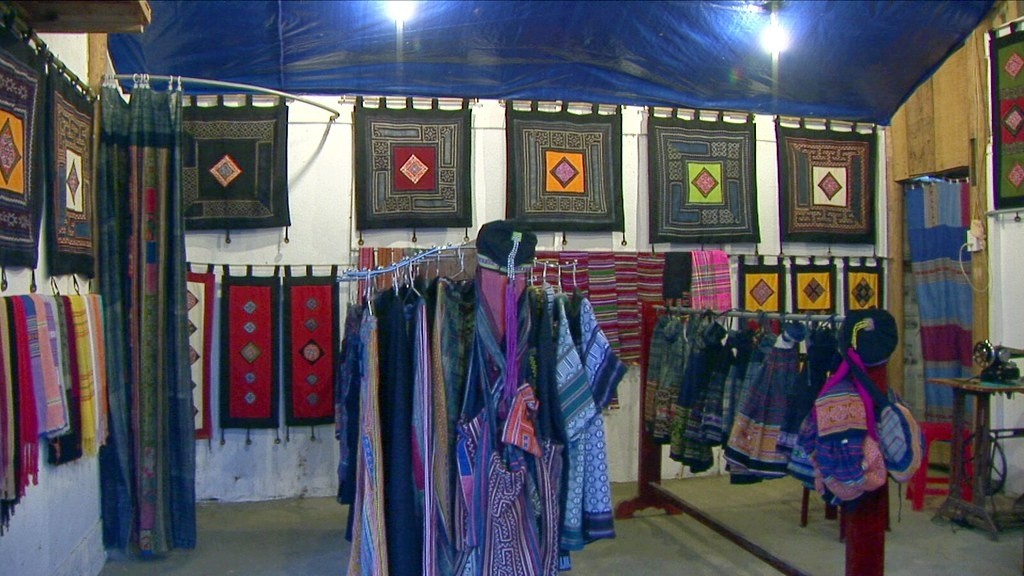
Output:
[476,220,537,399]
[837,308,898,365]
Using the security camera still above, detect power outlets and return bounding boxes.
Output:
[966,231,980,251]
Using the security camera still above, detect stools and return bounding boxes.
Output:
[906,422,972,511]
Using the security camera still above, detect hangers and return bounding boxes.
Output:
[346,243,578,317]
